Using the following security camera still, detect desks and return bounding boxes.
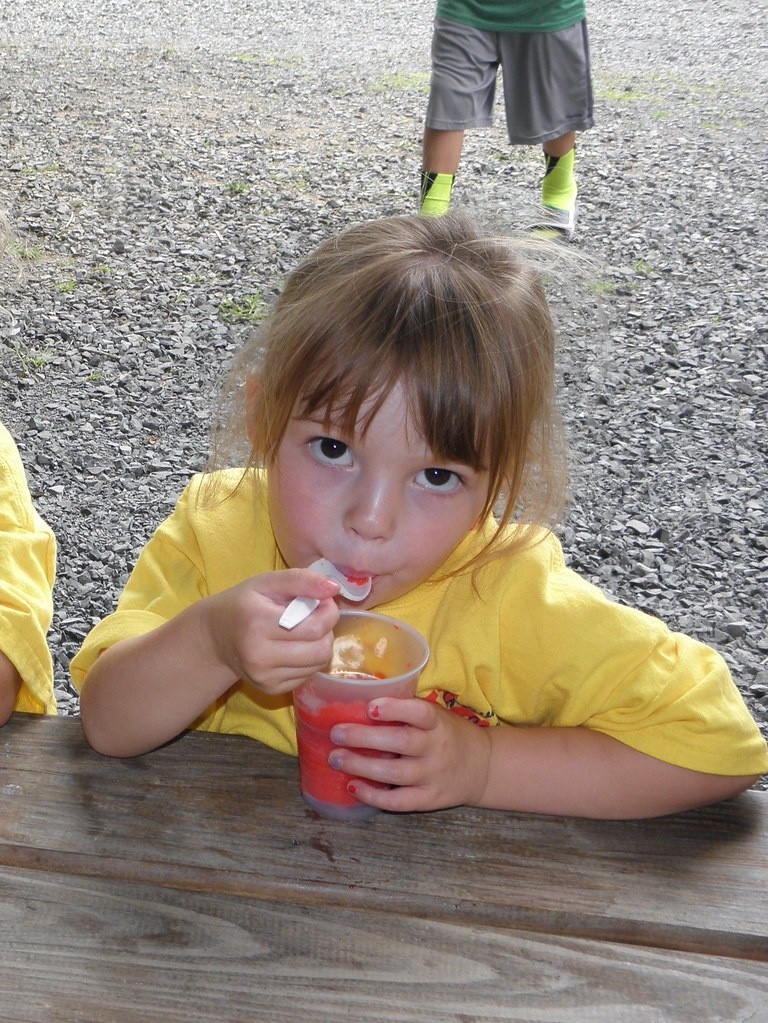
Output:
[0,709,767,1023]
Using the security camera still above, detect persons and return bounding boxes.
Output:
[414,0,597,249]
[0,416,58,734]
[68,205,766,822]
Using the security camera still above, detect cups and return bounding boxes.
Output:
[294,611,431,823]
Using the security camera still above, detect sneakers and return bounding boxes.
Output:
[527,205,578,269]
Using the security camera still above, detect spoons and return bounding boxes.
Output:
[278,558,372,630]
